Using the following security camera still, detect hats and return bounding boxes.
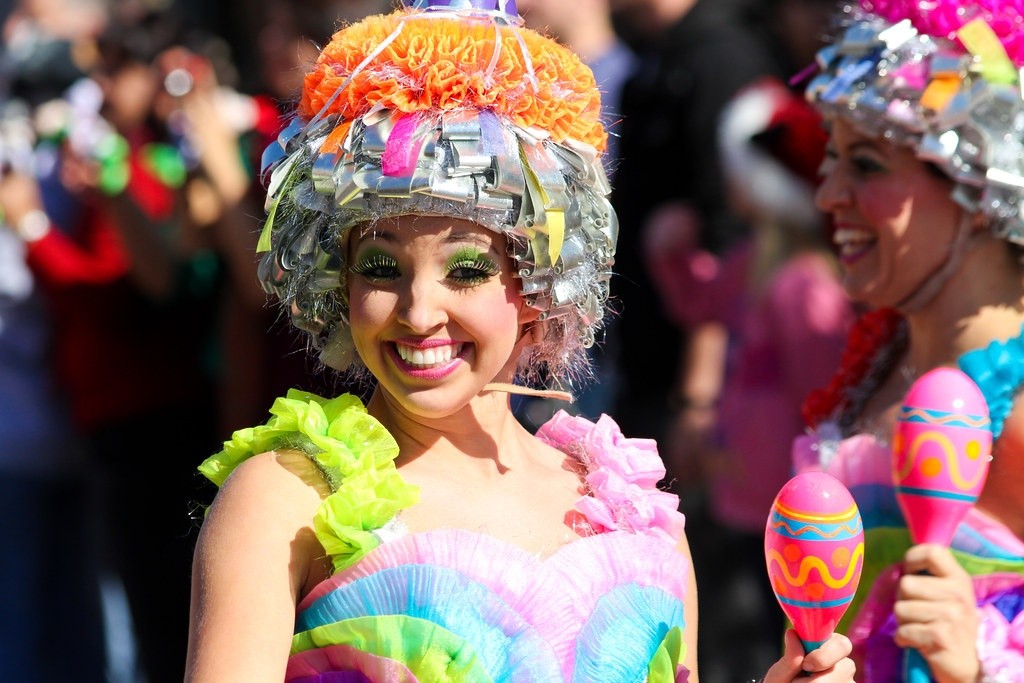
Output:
[710,72,835,232]
[243,0,621,394]
[786,0,1024,246]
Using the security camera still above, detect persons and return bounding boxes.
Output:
[517,0,855,683]
[792,0,1024,683]
[0,24,283,683]
[182,0,856,683]
